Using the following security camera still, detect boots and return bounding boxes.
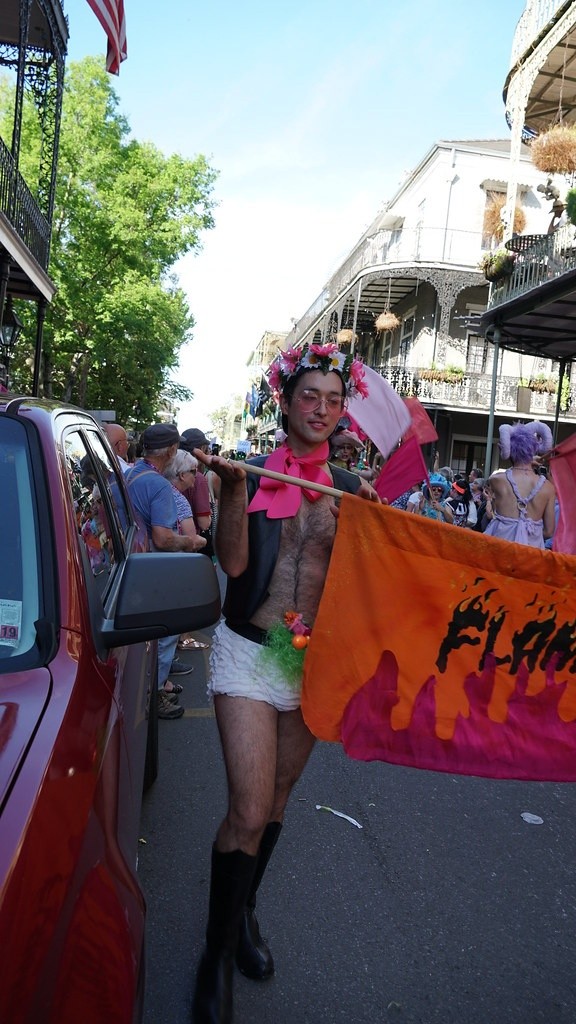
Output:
[195,840,258,1024]
[236,820,283,980]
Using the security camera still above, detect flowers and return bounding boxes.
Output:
[268,341,369,401]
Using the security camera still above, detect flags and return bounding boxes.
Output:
[548,448,576,555]
[373,435,426,505]
[346,360,411,458]
[401,397,438,444]
[86,0,127,76]
[243,375,272,418]
[557,433,576,475]
[342,411,368,441]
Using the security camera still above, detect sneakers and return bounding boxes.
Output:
[159,690,178,704]
[145,694,184,719]
[173,655,180,661]
[168,660,194,675]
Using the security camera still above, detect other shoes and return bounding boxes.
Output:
[164,682,183,693]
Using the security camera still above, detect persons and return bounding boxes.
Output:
[334,421,560,549]
[102,420,260,720]
[194,344,389,1024]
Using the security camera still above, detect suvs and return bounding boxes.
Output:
[0,395,222,1024]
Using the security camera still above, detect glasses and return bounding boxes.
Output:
[115,436,133,446]
[176,469,196,476]
[287,390,349,417]
[432,487,443,492]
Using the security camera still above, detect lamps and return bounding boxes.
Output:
[0,305,24,346]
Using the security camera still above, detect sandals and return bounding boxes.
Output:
[178,638,209,650]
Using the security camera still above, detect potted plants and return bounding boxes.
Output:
[478,252,516,282]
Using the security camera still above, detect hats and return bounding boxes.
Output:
[423,476,449,501]
[140,424,187,449]
[180,428,210,449]
[331,429,363,448]
